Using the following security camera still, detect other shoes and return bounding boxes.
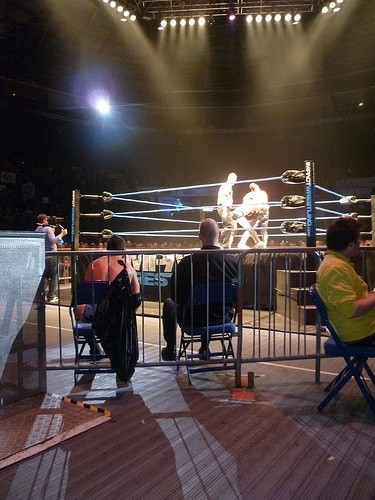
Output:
[87,338,102,354]
[161,346,177,361]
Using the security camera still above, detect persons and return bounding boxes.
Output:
[216,172,237,249]
[45,184,372,286]
[36,213,68,303]
[316,216,375,348]
[73,236,140,359]
[162,219,246,361]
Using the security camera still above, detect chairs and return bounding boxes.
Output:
[310,284,375,416]
[177,283,238,385]
[69,281,116,386]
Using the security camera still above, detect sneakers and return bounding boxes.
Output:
[49,297,59,303]
